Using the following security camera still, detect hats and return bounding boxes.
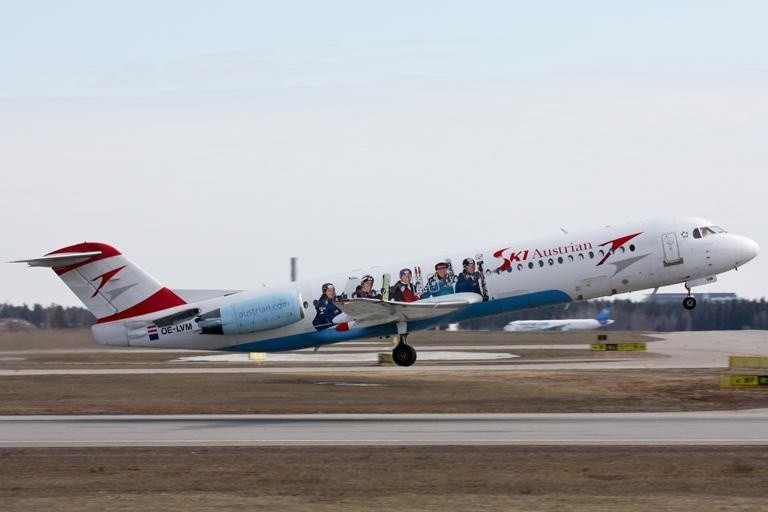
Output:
[435,257,450,268]
[400,268,412,278]
[360,275,374,284]
[322,282,335,294]
[462,257,475,269]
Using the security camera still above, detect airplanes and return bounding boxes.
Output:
[0,211,763,375]
[498,302,616,334]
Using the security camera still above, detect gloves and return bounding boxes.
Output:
[482,295,489,300]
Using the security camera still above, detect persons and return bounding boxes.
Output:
[419,258,455,299]
[390,268,418,302]
[310,282,348,331]
[455,257,481,294]
[350,275,384,301]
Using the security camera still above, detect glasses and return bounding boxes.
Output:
[437,266,445,270]
